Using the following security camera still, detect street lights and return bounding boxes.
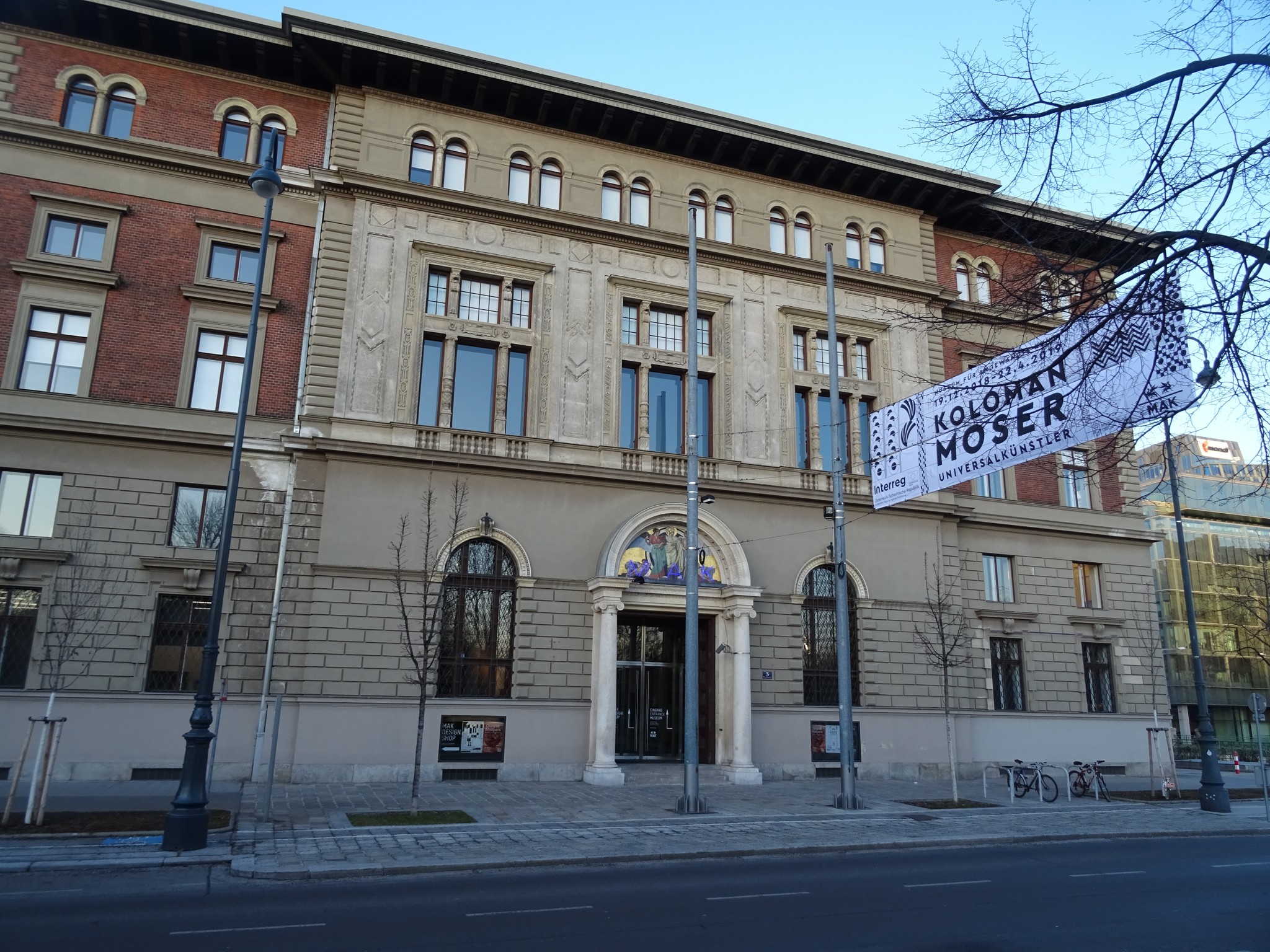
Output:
[1164,336,1232,813]
[162,126,287,852]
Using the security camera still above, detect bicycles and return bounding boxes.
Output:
[1068,760,1111,802]
[1007,759,1058,802]
